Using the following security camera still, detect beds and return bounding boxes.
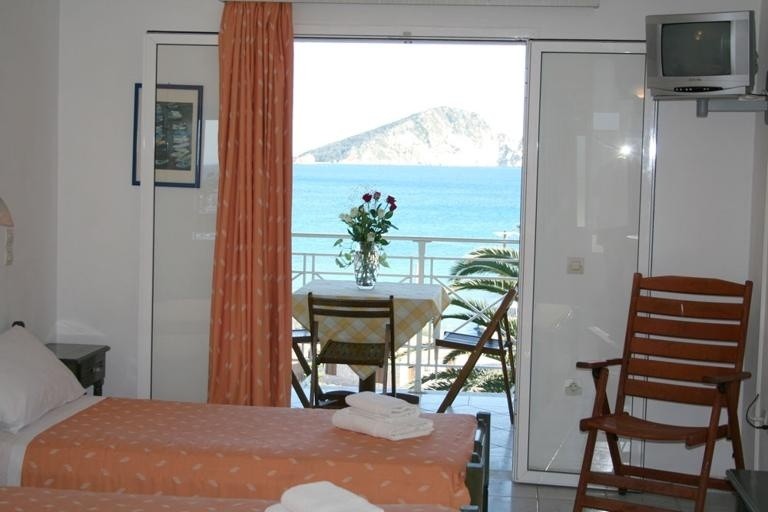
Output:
[0,394,492,512]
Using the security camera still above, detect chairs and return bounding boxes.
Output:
[291,280,518,426]
[576,273,753,512]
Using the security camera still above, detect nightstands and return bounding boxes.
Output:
[44,343,111,396]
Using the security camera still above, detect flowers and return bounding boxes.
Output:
[333,191,398,286]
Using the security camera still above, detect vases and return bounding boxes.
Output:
[353,241,380,291]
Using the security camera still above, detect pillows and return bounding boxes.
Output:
[1,325,88,435]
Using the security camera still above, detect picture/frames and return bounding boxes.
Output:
[131,82,203,189]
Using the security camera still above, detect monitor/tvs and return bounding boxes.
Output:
[645,11,755,99]
